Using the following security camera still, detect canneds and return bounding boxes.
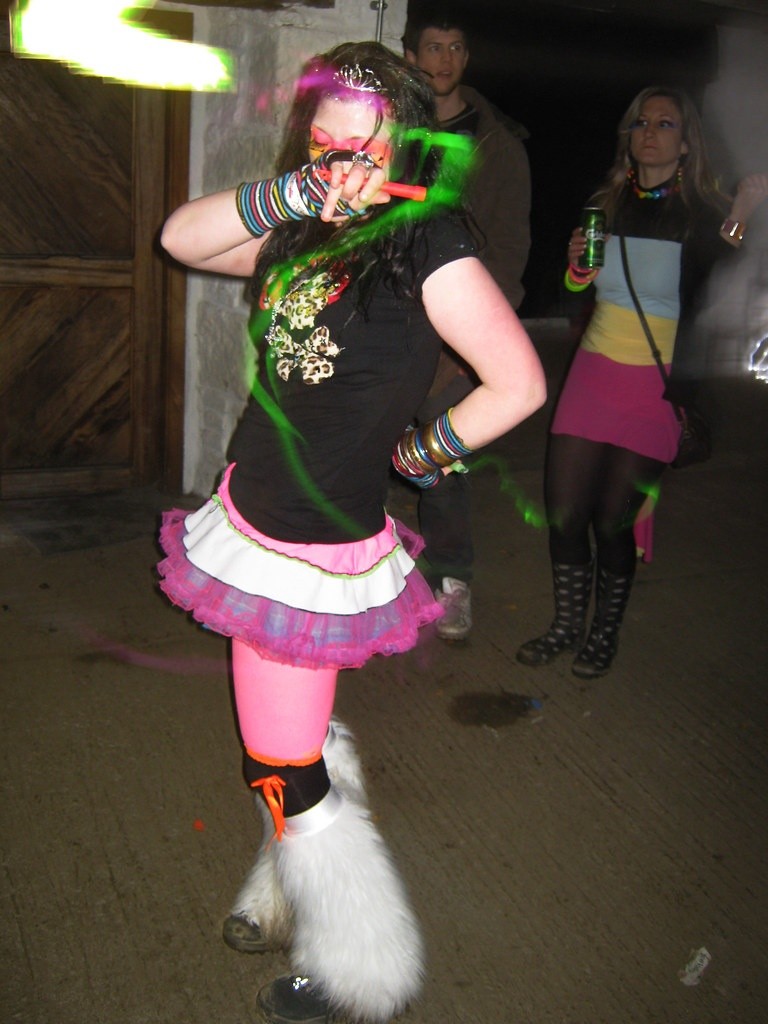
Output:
[578,207,606,269]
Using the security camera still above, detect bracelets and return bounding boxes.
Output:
[393,407,473,476]
[720,217,746,242]
[569,261,600,286]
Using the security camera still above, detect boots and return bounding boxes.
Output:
[570,563,633,678]
[517,548,595,667]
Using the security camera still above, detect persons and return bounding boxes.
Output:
[160,42,545,1023]
[400,17,532,641]
[517,85,767,679]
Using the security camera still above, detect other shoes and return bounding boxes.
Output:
[254,972,349,1024]
[224,912,289,954]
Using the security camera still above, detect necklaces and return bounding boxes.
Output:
[628,166,690,199]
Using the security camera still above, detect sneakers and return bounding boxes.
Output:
[433,576,473,638]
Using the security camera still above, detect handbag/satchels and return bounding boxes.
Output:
[668,395,709,470]
